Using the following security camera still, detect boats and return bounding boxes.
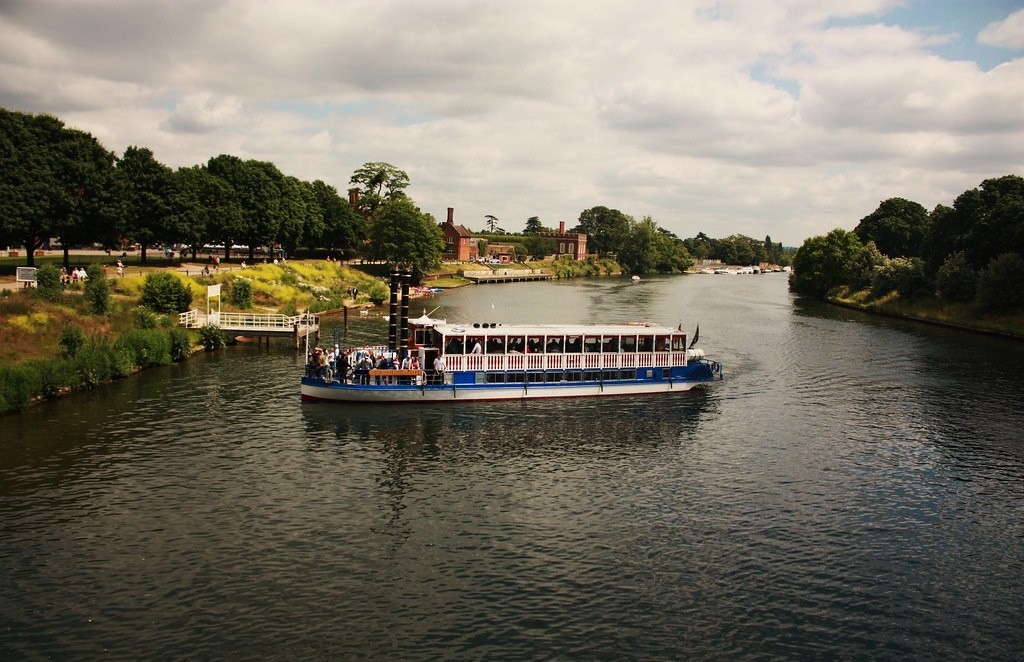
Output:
[632,276,640,280]
[300,314,723,401]
[701,265,791,274]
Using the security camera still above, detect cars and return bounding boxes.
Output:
[476,257,488,263]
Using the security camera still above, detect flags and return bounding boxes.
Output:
[690,326,699,348]
[673,324,681,343]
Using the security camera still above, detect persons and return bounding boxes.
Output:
[433,354,446,374]
[60,267,88,284]
[326,256,336,263]
[24,281,31,288]
[180,247,188,257]
[471,338,613,354]
[241,261,246,269]
[102,263,109,274]
[309,344,419,385]
[263,256,285,265]
[204,256,220,274]
[348,287,358,300]
[166,251,173,259]
[360,259,364,264]
[117,260,124,274]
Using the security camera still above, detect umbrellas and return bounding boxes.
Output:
[165,249,171,254]
